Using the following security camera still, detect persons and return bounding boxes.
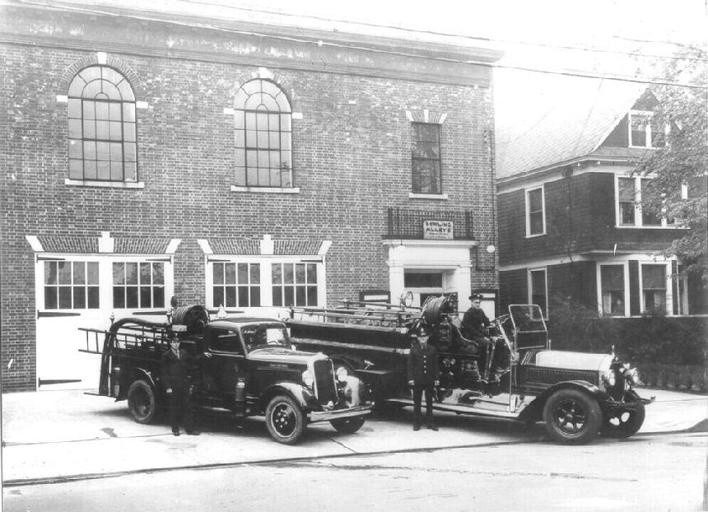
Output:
[406,326,440,431]
[460,293,497,384]
[160,333,213,435]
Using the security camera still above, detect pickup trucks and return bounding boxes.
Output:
[99,305,381,448]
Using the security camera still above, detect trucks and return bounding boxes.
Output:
[277,284,659,445]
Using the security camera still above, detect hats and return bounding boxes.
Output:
[468,294,485,301]
[416,326,430,337]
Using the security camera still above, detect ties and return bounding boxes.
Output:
[422,344,427,352]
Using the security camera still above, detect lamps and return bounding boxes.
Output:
[486,236,495,253]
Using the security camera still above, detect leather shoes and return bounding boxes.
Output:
[412,419,441,432]
[173,428,200,435]
